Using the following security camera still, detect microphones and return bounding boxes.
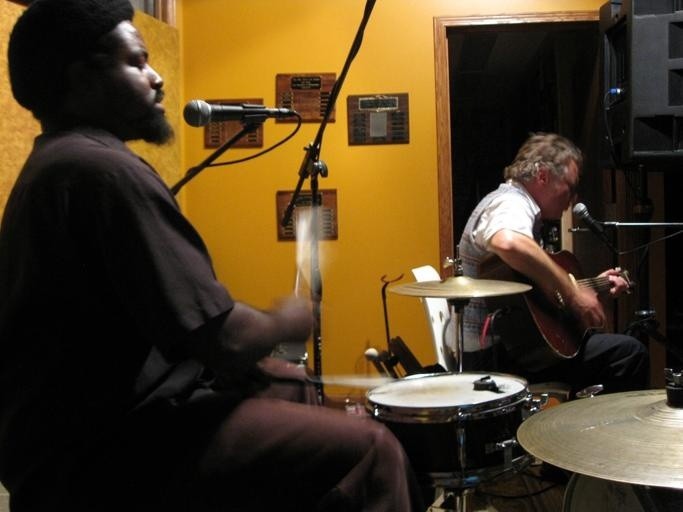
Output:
[573,203,617,251]
[364,348,393,379]
[183,99,295,127]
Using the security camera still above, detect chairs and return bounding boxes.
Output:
[409,264,569,406]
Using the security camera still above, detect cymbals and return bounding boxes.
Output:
[388,277,532,298]
[517,390,683,492]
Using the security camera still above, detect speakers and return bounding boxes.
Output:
[599,0,683,170]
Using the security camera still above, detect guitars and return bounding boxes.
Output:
[478,250,634,376]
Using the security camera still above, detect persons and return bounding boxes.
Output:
[450,132,651,393]
[0,1,411,512]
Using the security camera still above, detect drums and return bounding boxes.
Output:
[561,472,672,510]
[363,370,536,488]
